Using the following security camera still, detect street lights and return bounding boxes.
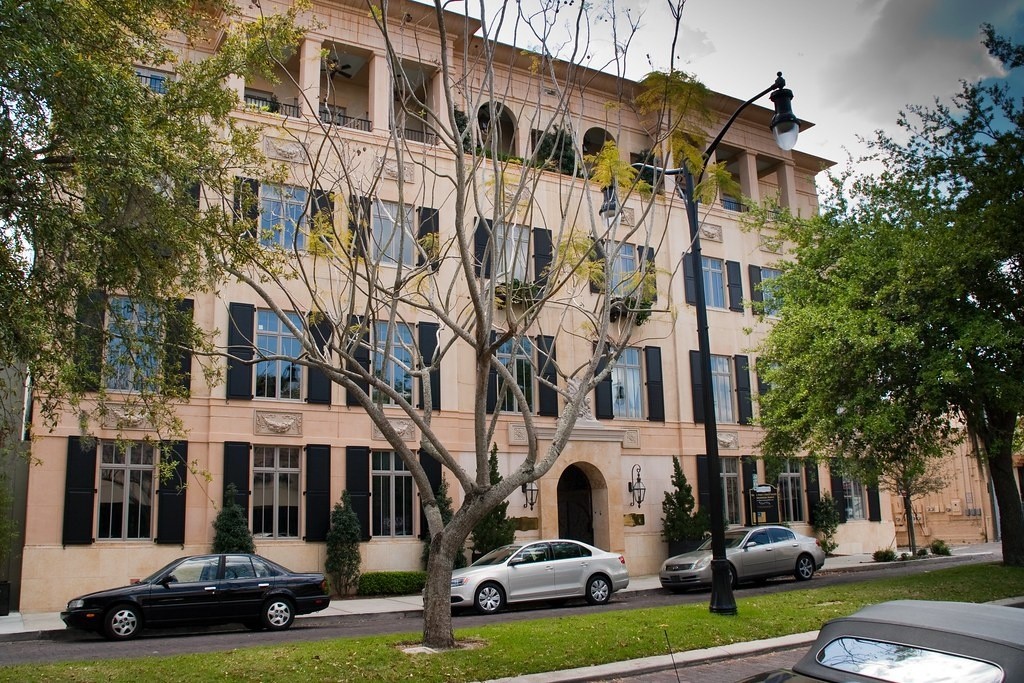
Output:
[598,71,802,617]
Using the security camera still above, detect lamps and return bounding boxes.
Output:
[522,481,538,511]
[628,464,646,509]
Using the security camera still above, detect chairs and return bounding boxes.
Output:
[535,549,546,561]
[523,550,534,563]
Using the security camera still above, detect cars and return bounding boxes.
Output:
[721,599,1024,683]
[59,554,330,642]
[422,539,630,616]
[658,525,827,593]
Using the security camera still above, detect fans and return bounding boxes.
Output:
[321,59,352,81]
